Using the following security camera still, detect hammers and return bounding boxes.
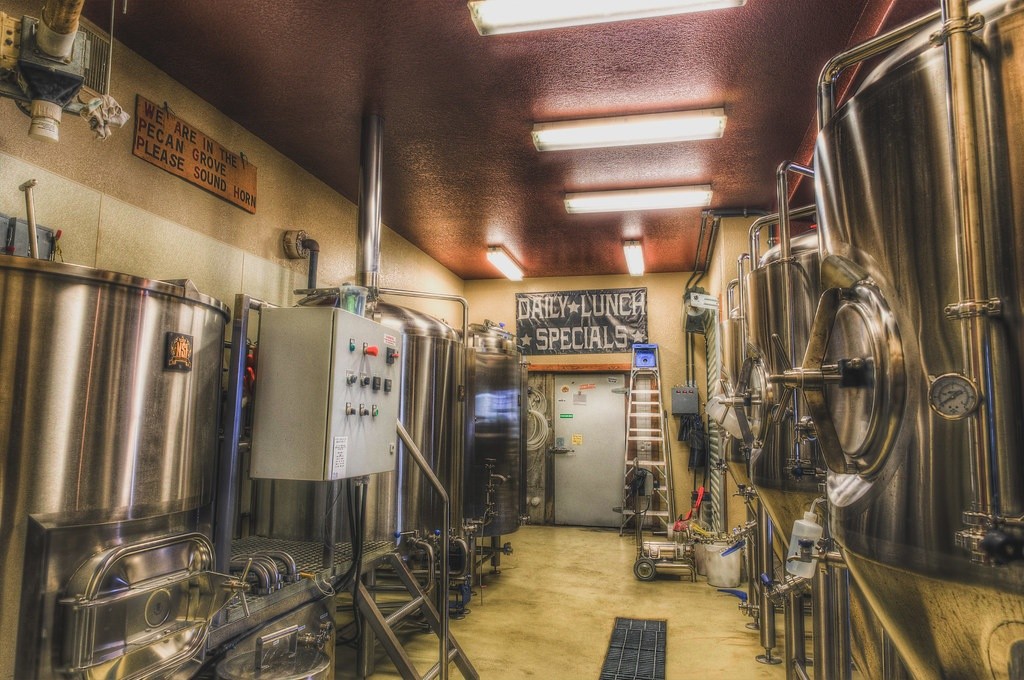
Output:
[18,178,40,260]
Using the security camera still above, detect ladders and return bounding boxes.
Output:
[619,343,676,537]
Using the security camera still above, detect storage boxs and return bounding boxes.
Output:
[695,542,710,577]
[704,541,741,588]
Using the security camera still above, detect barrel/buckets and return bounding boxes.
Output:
[704,542,741,587]
[695,542,705,576]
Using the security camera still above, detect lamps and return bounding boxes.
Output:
[486,244,526,282]
[625,239,645,277]
[563,185,714,213]
[467,1,746,35]
[531,107,729,152]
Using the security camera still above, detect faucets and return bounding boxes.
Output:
[393,529,425,552]
[739,602,760,629]
[732,483,755,504]
[712,462,722,472]
[787,539,830,564]
[433,529,457,552]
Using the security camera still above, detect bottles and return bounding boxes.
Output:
[786,500,824,579]
